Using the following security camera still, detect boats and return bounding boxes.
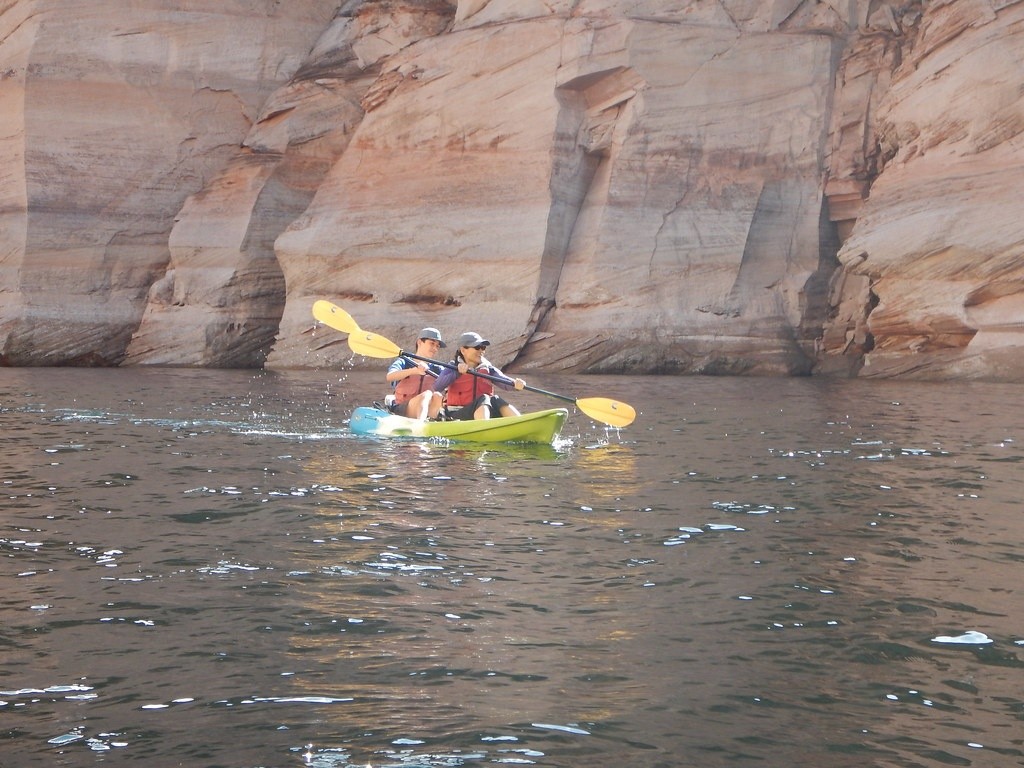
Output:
[349,401,568,447]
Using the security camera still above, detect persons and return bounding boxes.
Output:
[434,332,526,420]
[386,328,447,421]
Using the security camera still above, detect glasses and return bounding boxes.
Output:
[467,345,487,351]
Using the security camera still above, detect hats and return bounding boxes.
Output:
[416,327,446,348]
[458,332,491,348]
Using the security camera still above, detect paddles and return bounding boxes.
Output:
[346,329,635,428]
[312,300,441,378]
